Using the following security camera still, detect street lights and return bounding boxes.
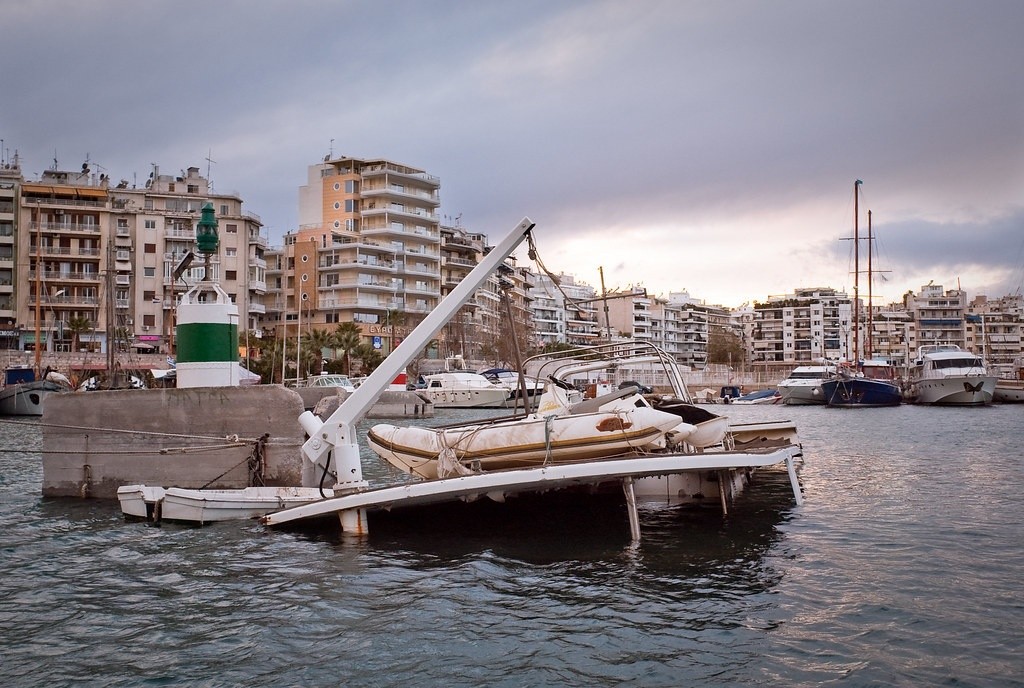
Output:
[835,322,848,366]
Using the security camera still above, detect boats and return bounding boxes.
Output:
[366,342,726,478]
[305,374,356,393]
[409,369,511,410]
[73,234,149,392]
[117,484,333,524]
[776,179,902,409]
[729,391,782,405]
[902,343,1000,405]
[479,369,580,407]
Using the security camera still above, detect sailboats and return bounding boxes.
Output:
[0,195,72,418]
